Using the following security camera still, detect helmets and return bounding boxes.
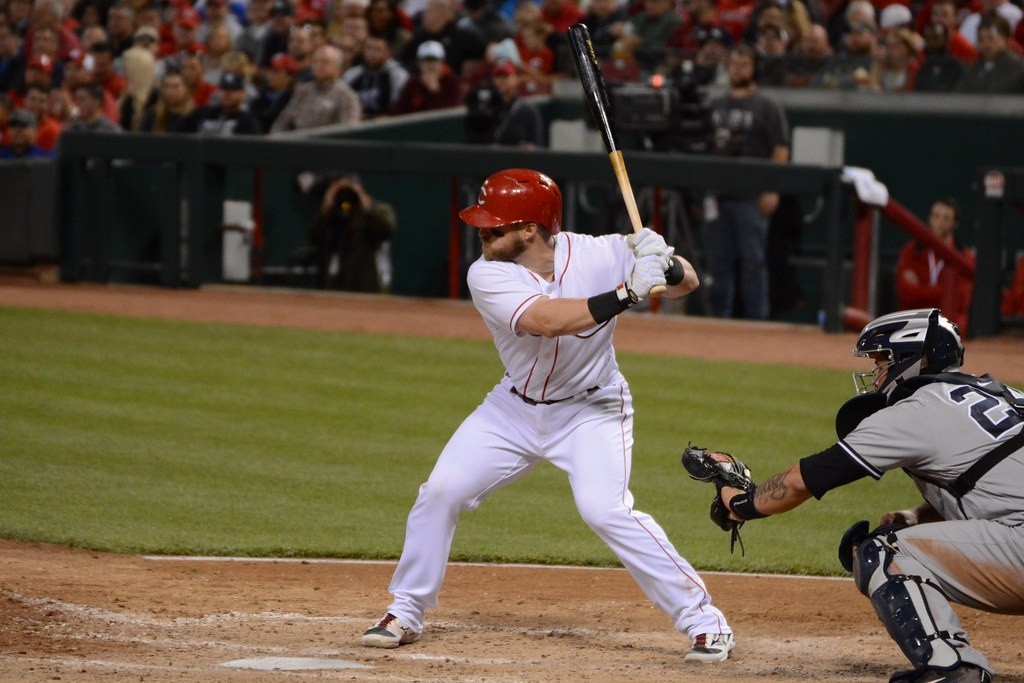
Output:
[852,307,964,400]
[458,170,561,236]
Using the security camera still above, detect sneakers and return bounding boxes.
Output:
[685,634,735,666]
[362,613,419,648]
[890,663,991,683]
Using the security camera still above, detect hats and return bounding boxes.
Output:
[135,26,159,45]
[219,71,245,92]
[418,43,445,62]
[492,60,514,77]
[176,6,200,30]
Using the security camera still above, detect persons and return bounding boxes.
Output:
[363,170,736,664]
[0,0,1024,340]
[681,306,1024,683]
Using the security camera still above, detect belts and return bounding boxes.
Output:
[513,384,600,406]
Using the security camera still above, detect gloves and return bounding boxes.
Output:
[626,227,684,287]
[615,254,670,306]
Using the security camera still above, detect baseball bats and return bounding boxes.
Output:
[566,21,668,300]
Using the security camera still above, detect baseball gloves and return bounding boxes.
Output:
[677,439,757,559]
